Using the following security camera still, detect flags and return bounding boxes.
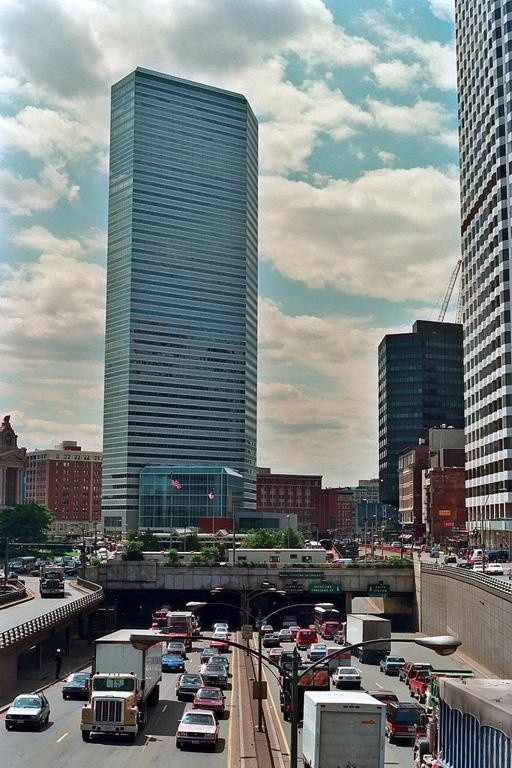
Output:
[170,477,182,490]
[208,492,214,501]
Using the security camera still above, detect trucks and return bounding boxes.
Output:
[38,564,65,599]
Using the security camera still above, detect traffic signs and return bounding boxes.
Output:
[368,584,390,596]
[310,584,341,593]
[279,572,324,579]
[285,584,303,593]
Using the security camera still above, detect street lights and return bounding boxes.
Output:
[286,514,290,548]
[184,598,334,737]
[207,580,287,655]
[361,497,367,564]
[118,519,124,540]
[69,523,86,579]
[89,520,97,557]
[481,487,507,572]
[122,630,464,768]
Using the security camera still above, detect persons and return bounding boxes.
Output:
[106,542,116,553]
[4,563,15,575]
[448,549,452,556]
[55,648,62,679]
[119,679,129,689]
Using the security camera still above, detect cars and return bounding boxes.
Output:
[304,537,421,564]
[6,604,232,752]
[421,544,510,575]
[0,534,124,585]
